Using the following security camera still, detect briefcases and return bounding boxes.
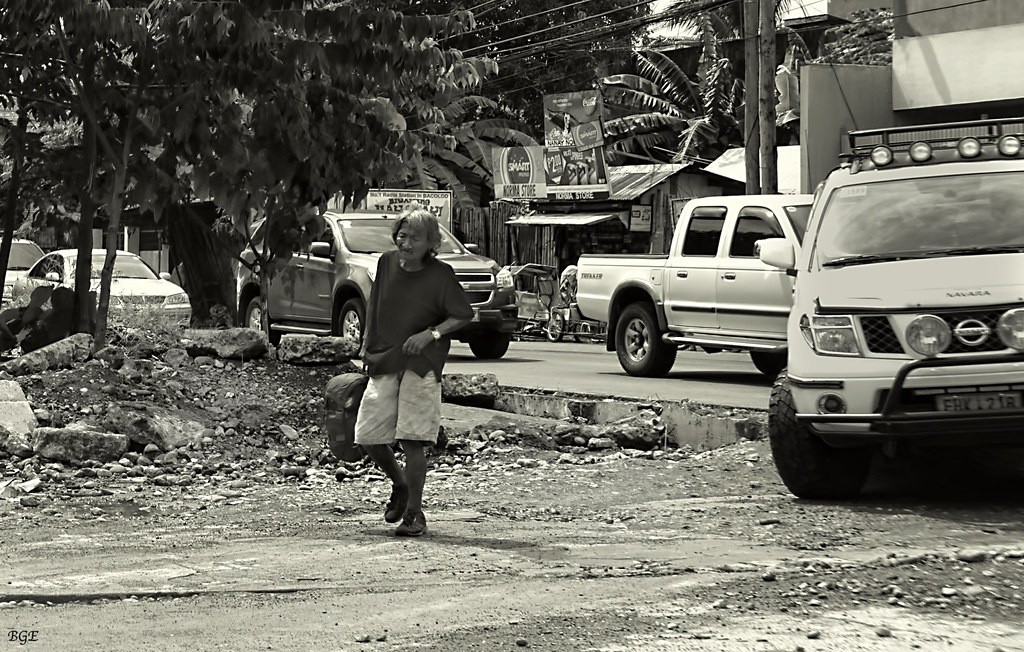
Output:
[323,363,370,462]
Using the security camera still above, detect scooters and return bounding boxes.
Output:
[505,259,599,344]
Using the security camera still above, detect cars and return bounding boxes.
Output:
[238,207,517,361]
[16,247,192,336]
[1,237,47,312]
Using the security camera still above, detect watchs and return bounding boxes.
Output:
[428,325,441,342]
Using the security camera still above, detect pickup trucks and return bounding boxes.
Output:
[575,195,828,379]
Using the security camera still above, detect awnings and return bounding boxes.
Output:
[505,211,615,228]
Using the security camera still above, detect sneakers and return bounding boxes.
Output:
[383,483,409,523]
[394,509,428,538]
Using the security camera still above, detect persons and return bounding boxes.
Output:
[354,204,475,536]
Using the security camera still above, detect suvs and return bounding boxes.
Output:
[768,115,1024,504]
